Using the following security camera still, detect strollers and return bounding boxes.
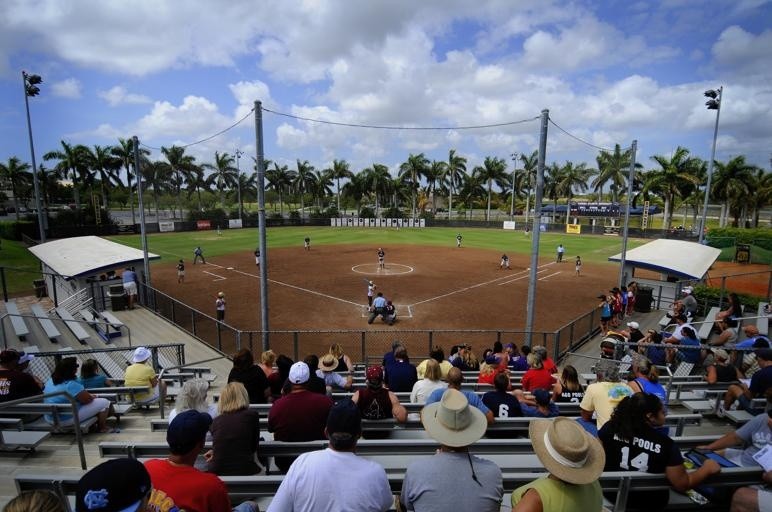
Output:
[590,334,626,376]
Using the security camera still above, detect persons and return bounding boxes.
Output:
[368,281,396,325]
[1,348,166,436]
[303,235,311,252]
[175,259,187,284]
[1,488,72,512]
[193,246,207,265]
[253,247,261,271]
[574,255,583,277]
[456,233,464,248]
[595,281,772,397]
[216,292,226,330]
[556,244,565,264]
[78,343,772,512]
[376,247,386,269]
[121,267,139,311]
[524,225,530,236]
[499,253,513,271]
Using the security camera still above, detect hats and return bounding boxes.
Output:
[681,286,692,293]
[503,342,513,347]
[420,389,488,448]
[134,347,150,363]
[366,366,383,386]
[167,411,212,454]
[289,361,310,384]
[534,389,550,400]
[318,353,338,373]
[529,417,607,485]
[329,397,361,437]
[18,352,34,364]
[710,347,729,359]
[627,322,639,329]
[75,459,151,512]
[598,295,607,299]
[218,291,226,298]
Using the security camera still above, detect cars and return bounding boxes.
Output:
[436,208,449,212]
[0,204,89,217]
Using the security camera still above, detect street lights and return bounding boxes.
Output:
[231,146,246,219]
[508,150,519,220]
[20,69,47,242]
[695,84,723,242]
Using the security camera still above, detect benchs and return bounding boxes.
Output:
[1,354,377,512]
[375,300,772,511]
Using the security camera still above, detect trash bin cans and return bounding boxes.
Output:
[735,244,751,264]
[634,287,655,313]
[107,283,126,312]
